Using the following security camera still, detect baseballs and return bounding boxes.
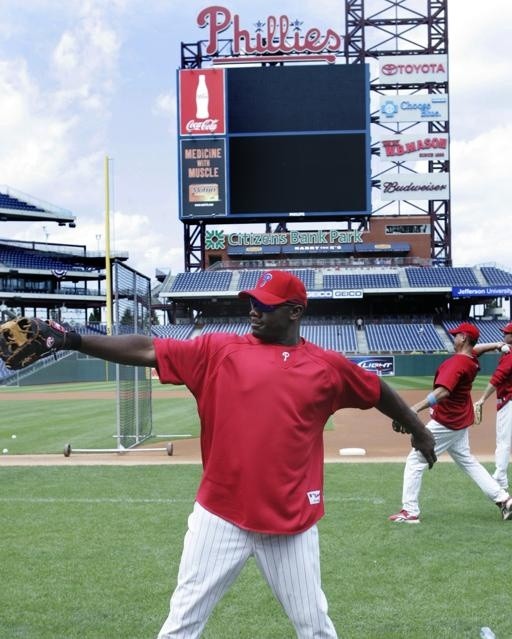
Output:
[501,344,511,354]
[11,435,17,440]
[3,449,9,454]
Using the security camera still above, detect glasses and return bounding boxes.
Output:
[250,297,296,312]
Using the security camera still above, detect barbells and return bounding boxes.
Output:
[64,442,173,457]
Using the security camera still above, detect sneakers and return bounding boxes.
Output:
[492,474,508,489]
[496,496,512,520]
[390,508,420,524]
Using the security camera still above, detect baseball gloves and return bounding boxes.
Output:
[473,399,484,425]
[392,407,417,434]
[0,316,81,370]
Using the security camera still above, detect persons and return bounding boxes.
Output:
[1,267,437,638]
[386,320,512,524]
[473,322,512,490]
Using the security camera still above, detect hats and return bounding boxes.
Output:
[239,271,307,305]
[448,322,480,338]
[501,323,512,333]
